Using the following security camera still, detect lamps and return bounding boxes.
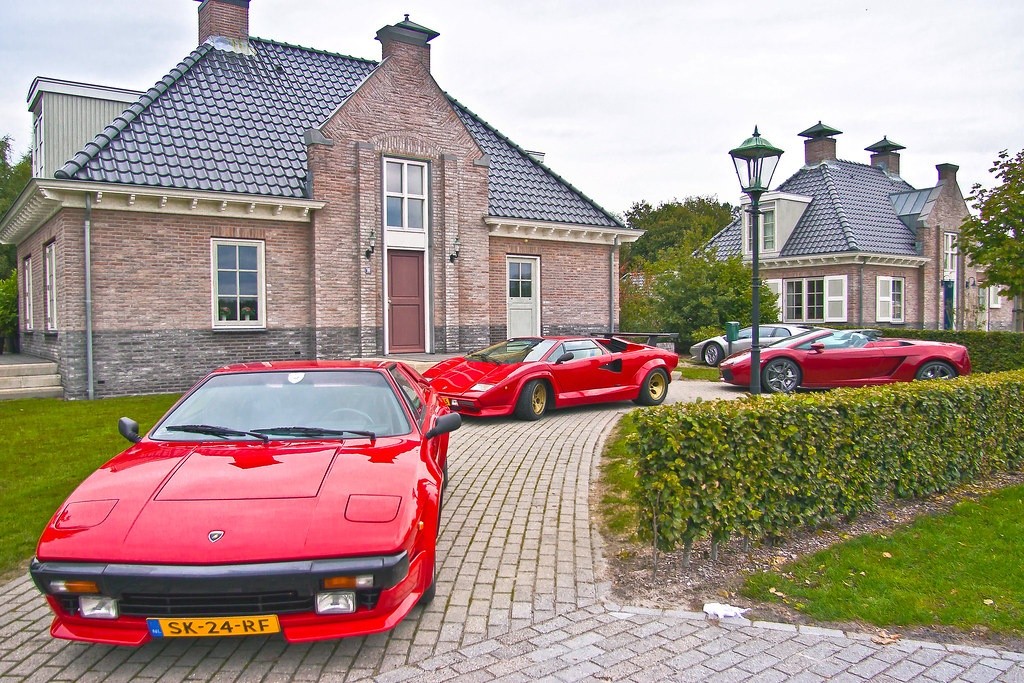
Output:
[939,274,953,289]
[448,234,460,263]
[364,228,376,260]
[966,276,977,290]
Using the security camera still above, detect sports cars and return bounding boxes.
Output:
[719,330,976,394]
[28,358,462,647]
[690,321,837,367]
[413,337,679,422]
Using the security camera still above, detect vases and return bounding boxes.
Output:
[244,315,250,321]
[220,315,226,321]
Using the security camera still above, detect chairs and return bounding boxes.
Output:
[841,332,868,348]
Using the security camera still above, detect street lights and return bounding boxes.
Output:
[725,124,791,397]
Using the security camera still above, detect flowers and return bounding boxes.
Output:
[219,307,231,315]
[240,306,255,316]
